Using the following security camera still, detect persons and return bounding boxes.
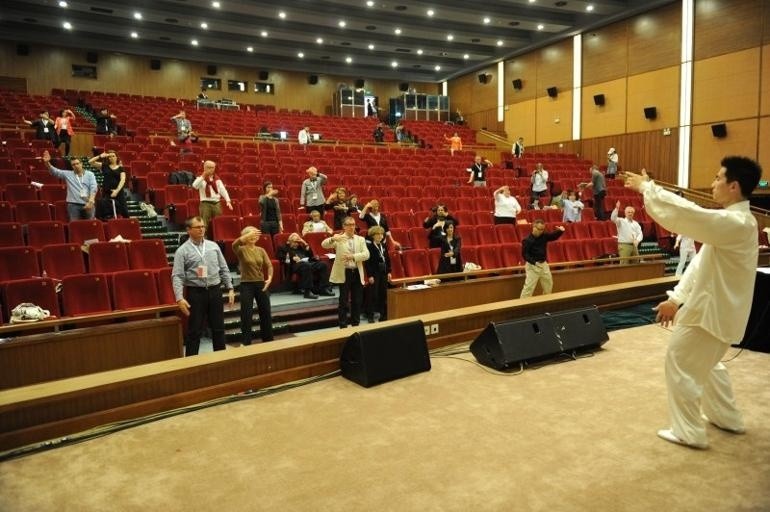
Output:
[373,127,384,141]
[43,150,99,220]
[529,163,549,209]
[467,156,493,187]
[53,109,76,156]
[611,201,644,264]
[199,89,206,98]
[172,216,234,357]
[551,166,607,222]
[623,154,763,449]
[170,110,192,152]
[605,147,619,178]
[396,123,408,141]
[673,235,697,276]
[191,160,233,239]
[445,132,462,156]
[423,204,464,284]
[22,110,58,144]
[96,107,112,135]
[298,127,312,144]
[88,149,129,218]
[512,138,525,158]
[493,186,522,225]
[258,182,283,238]
[232,226,273,348]
[277,166,400,329]
[520,220,565,297]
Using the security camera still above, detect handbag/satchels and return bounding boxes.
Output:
[9,302,50,324]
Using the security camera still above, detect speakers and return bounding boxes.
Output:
[86,52,99,63]
[547,86,557,97]
[711,123,727,138]
[150,59,161,70]
[594,94,605,105]
[17,45,30,55]
[208,64,217,75]
[340,319,431,388]
[258,72,269,79]
[478,74,487,83]
[512,80,522,89]
[309,76,318,84]
[644,107,656,119]
[355,80,364,87]
[399,83,408,91]
[469,305,610,370]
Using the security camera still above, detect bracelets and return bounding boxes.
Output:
[99,154,101,159]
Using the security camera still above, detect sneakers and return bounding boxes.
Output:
[658,429,687,445]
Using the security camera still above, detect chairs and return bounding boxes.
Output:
[1,87,769,338]
